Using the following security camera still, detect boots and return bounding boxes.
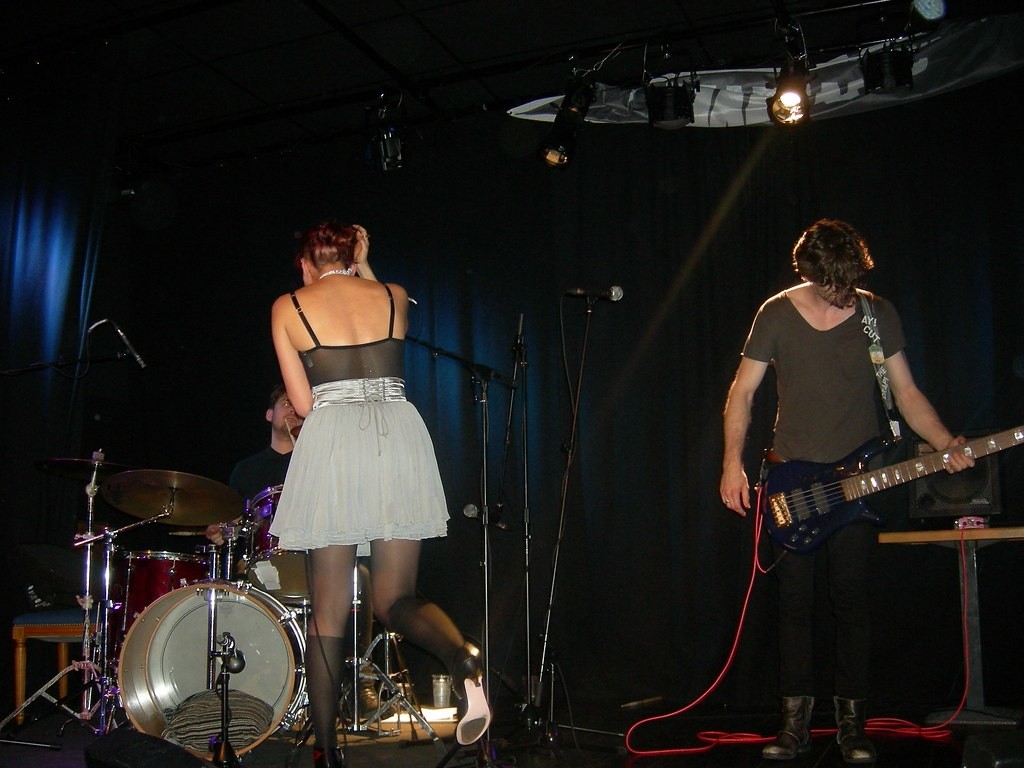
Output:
[833,695,878,765]
[761,695,815,761]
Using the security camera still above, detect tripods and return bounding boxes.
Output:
[0,465,177,737]
[287,298,631,768]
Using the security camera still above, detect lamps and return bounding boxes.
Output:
[857,49,914,93]
[534,108,586,174]
[375,124,412,173]
[765,66,817,131]
[643,84,695,131]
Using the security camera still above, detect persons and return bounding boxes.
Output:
[267,221,493,768]
[205,385,396,721]
[720,220,973,763]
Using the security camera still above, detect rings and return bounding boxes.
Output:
[726,500,730,503]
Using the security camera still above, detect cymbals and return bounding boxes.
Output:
[38,455,133,483]
[99,468,246,527]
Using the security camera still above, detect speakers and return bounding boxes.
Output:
[906,429,1001,519]
[84,727,220,768]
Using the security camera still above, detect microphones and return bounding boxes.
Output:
[111,322,147,369]
[227,650,245,674]
[566,286,624,301]
[463,504,510,530]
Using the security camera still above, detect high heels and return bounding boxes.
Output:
[312,745,346,768]
[449,642,493,746]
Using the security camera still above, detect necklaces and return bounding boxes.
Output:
[320,268,352,278]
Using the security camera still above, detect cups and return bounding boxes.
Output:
[432,674,453,708]
[398,682,414,713]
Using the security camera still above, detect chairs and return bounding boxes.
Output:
[10,542,105,726]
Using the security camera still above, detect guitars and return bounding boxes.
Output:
[759,425,1024,556]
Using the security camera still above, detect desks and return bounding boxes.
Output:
[877,525,1024,726]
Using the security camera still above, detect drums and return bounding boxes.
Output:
[104,548,223,696]
[245,482,313,607]
[117,580,307,758]
